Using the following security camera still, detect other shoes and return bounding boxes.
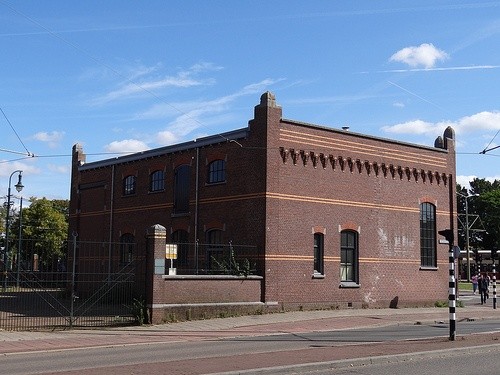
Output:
[473,292,475,294]
[484,300,486,303]
[481,301,483,305]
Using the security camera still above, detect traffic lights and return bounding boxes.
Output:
[437,229,454,243]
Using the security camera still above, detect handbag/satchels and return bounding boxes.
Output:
[486,288,490,299]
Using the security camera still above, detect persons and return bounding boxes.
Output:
[472,274,478,295]
[478,275,488,304]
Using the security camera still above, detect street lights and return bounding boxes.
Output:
[3,169,25,292]
[456,192,480,282]
